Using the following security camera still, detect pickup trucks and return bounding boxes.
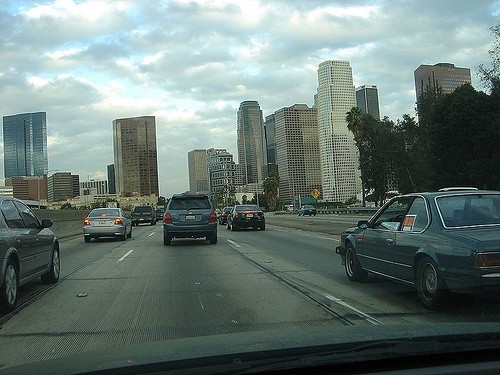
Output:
[131,205,156,227]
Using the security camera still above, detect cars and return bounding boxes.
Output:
[82,207,133,242]
[219,207,233,225]
[155,208,166,223]
[216,209,222,219]
[297,205,317,217]
[336,186,500,312]
[226,204,265,231]
[0,196,60,314]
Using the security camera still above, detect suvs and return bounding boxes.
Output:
[163,194,218,246]
